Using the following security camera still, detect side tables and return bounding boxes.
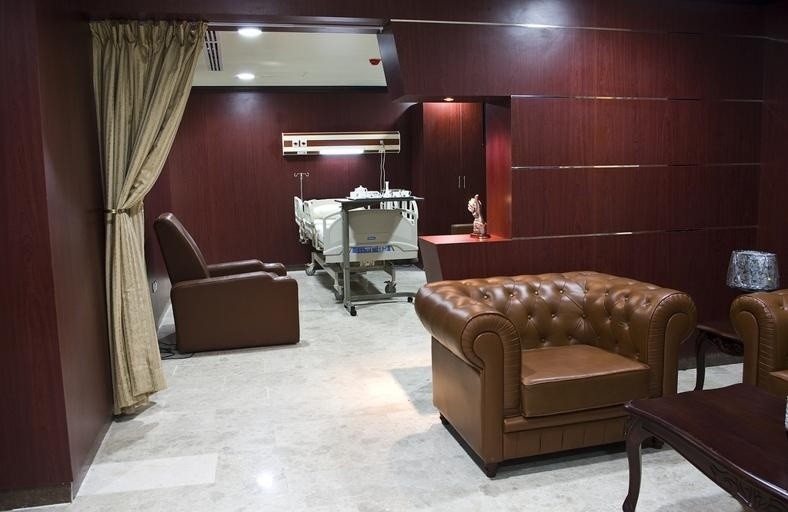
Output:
[694,317,744,392]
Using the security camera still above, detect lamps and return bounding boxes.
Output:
[725,247,781,294]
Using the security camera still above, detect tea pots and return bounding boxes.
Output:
[355,185,367,198]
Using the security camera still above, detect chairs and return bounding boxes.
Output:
[410,268,702,479]
[154,212,303,355]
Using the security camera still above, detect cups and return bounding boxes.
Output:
[350,191,411,198]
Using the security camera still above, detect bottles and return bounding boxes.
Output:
[383,182,392,197]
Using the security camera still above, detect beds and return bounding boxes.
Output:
[293,192,421,317]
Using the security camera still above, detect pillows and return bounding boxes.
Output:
[313,205,341,220]
